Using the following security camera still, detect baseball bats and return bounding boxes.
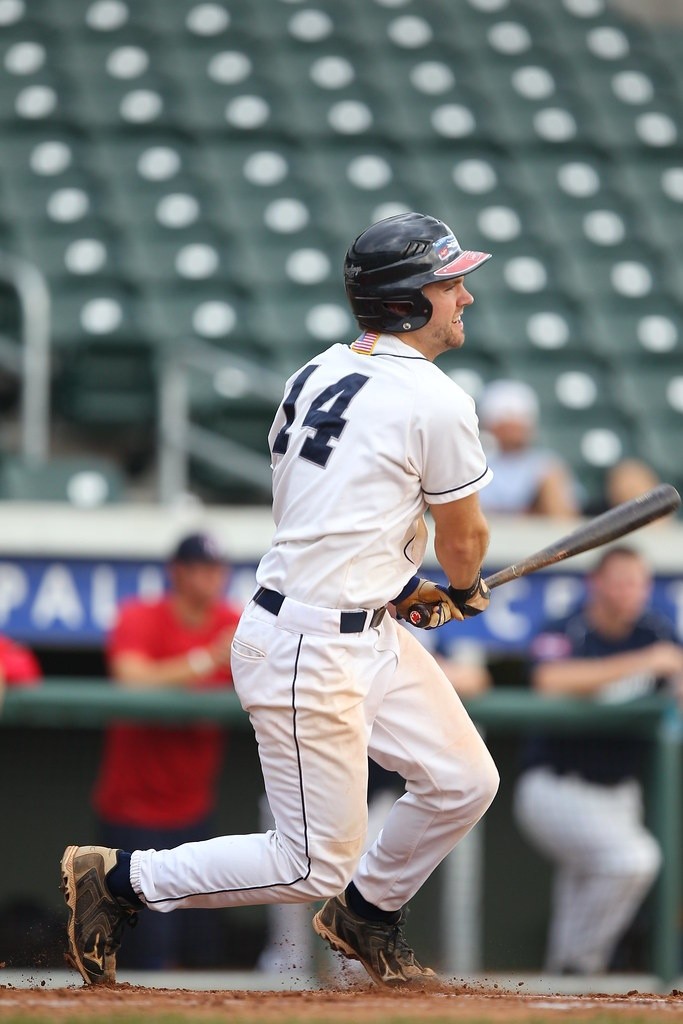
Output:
[408,481,682,627]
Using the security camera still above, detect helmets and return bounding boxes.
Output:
[344,208,493,332]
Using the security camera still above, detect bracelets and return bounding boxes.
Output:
[187,646,215,677]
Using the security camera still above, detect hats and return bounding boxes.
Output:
[176,532,228,568]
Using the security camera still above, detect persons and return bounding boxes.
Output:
[60,214,491,992]
[510,543,683,971]
[82,529,248,970]
[336,574,488,991]
[467,378,678,523]
[1,632,47,684]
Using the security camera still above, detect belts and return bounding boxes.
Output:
[251,587,385,634]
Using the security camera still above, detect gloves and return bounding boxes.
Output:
[449,565,493,620]
[397,580,464,631]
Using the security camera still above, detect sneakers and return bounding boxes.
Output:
[312,896,443,997]
[61,842,133,989]
